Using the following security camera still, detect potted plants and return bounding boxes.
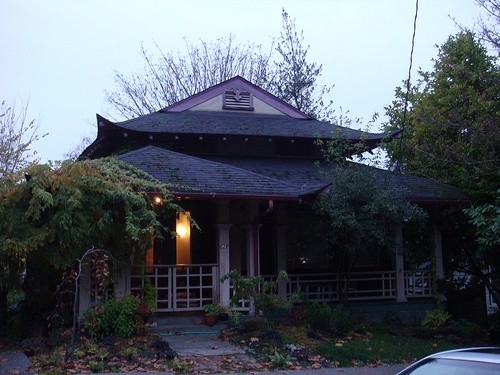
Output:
[203,303,219,327]
[292,292,306,310]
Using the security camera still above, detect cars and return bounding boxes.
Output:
[394,345,500,375]
